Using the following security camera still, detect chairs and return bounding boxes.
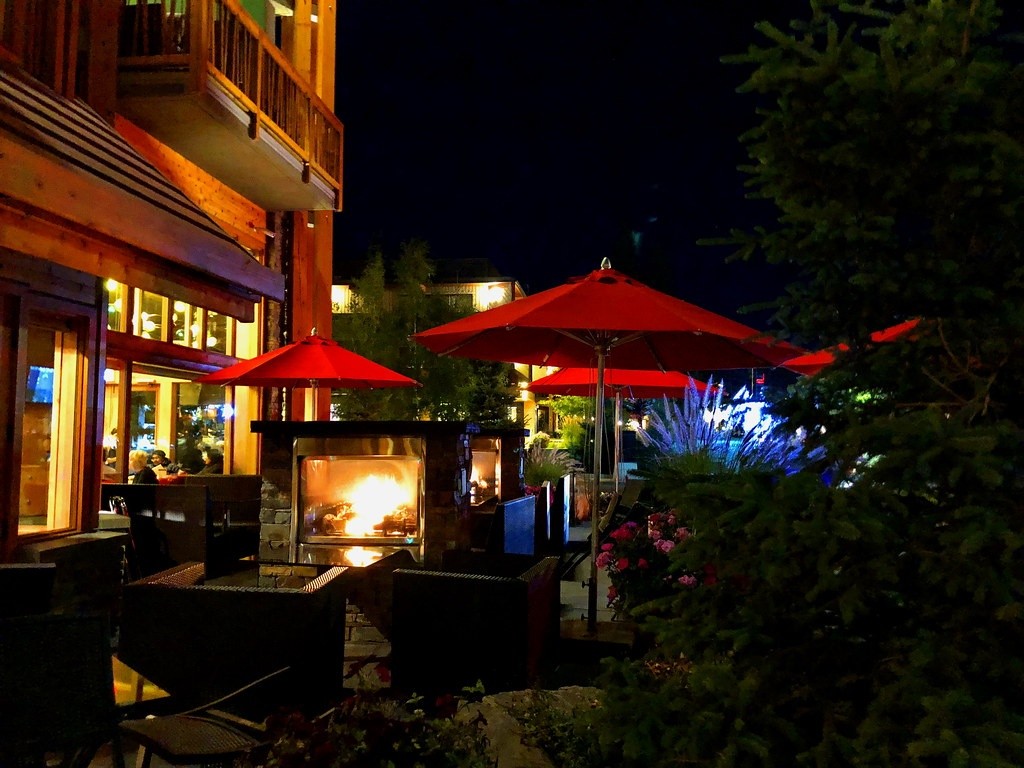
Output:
[392,550,562,694]
[123,561,349,720]
[536,481,551,542]
[0,563,126,768]
[118,666,336,768]
[550,473,571,546]
[471,495,537,556]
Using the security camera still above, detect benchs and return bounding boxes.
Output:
[185,474,263,559]
[100,483,226,579]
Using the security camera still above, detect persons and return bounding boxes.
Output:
[102,446,223,486]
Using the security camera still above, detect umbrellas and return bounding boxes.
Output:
[416,258,1024,635]
[194,327,422,420]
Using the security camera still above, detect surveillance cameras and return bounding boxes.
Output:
[258,228,276,238]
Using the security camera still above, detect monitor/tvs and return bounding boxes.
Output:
[25,364,54,404]
